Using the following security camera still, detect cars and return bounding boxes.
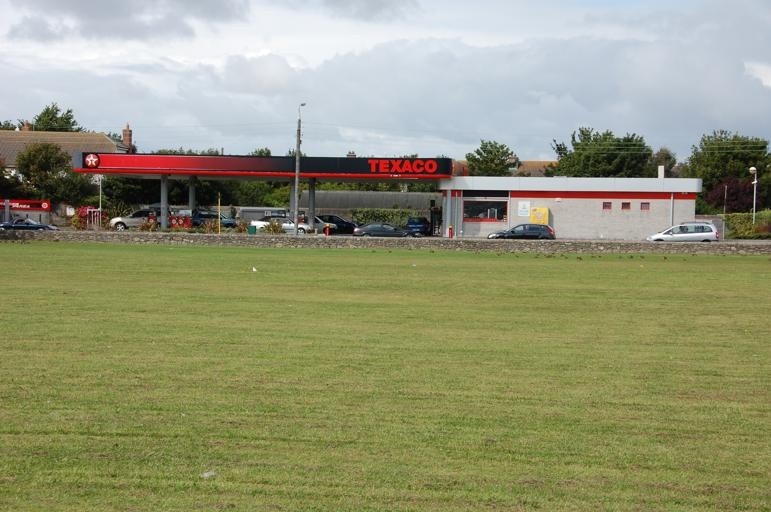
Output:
[250,214,431,237]
[0,217,59,231]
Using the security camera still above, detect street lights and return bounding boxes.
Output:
[748,166,758,225]
[294,103,306,236]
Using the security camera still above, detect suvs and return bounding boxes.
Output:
[646,221,719,243]
[487,223,556,239]
[110,208,236,232]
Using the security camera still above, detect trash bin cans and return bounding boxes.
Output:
[248,226,256,234]
